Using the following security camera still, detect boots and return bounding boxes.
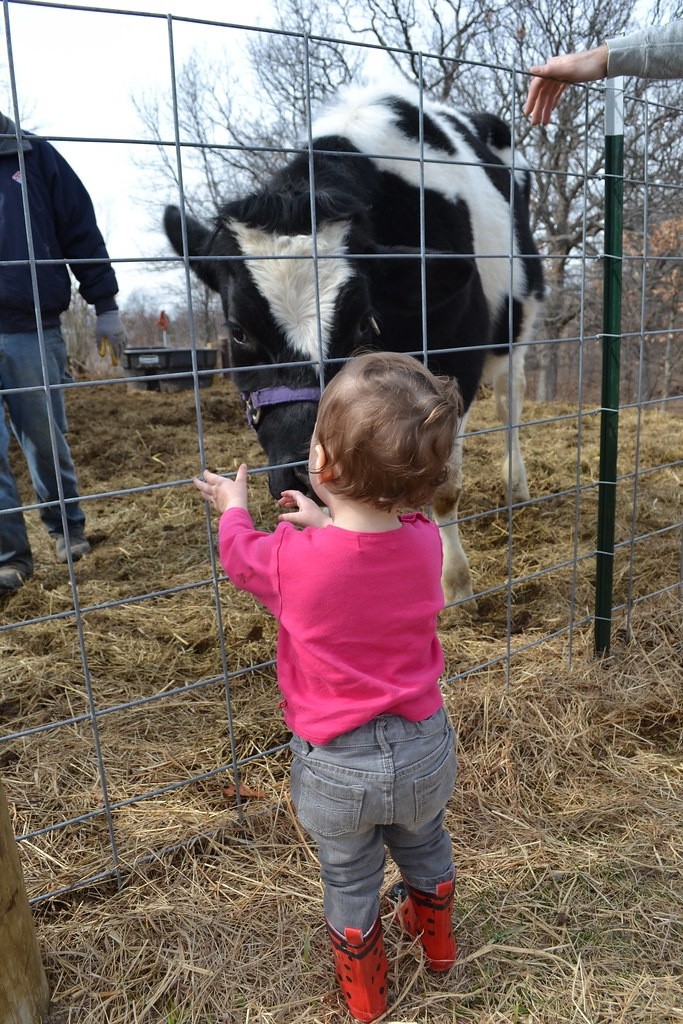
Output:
[387,870,456,970]
[323,914,388,1022]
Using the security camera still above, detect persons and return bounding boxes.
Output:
[194,351,464,1021]
[0,112,129,590]
[525,19,683,125]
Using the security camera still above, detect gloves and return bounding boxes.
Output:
[95,311,127,366]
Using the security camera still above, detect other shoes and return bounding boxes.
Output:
[55,534,92,564]
[0,565,32,594]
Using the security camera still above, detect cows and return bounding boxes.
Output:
[161,80,547,619]
